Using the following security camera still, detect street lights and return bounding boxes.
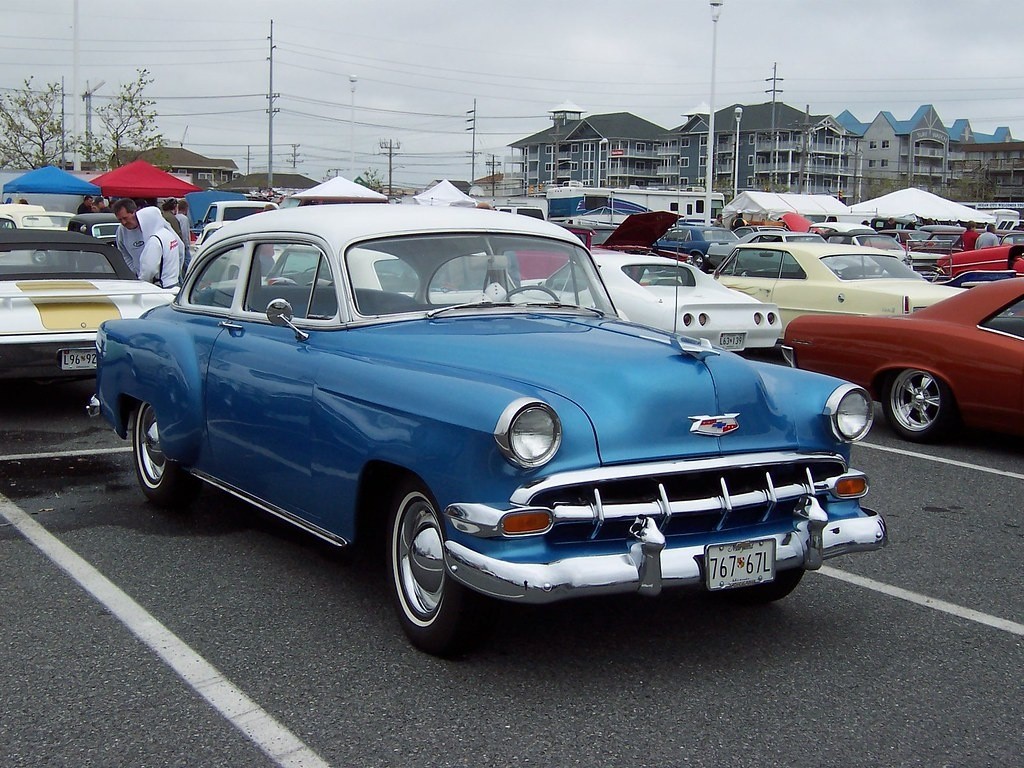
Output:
[348,74,359,184]
[704,0,726,242]
[732,107,744,197]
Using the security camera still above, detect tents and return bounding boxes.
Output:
[721,186,850,230]
[848,187,997,227]
[291,176,388,207]
[87,159,204,198]
[413,179,476,208]
[2,165,101,206]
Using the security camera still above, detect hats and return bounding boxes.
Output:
[861,221,871,224]
[162,197,176,207]
[92,197,103,206]
[737,209,741,213]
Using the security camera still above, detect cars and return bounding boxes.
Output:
[0,201,1024,447]
[85,206,892,656]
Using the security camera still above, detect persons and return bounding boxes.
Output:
[730,209,749,231]
[77,196,197,299]
[257,204,278,277]
[714,213,725,229]
[962,221,980,251]
[974,223,1000,249]
[883,217,935,230]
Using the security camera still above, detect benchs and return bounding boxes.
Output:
[198,284,425,319]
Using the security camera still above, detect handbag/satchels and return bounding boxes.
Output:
[153,277,164,289]
